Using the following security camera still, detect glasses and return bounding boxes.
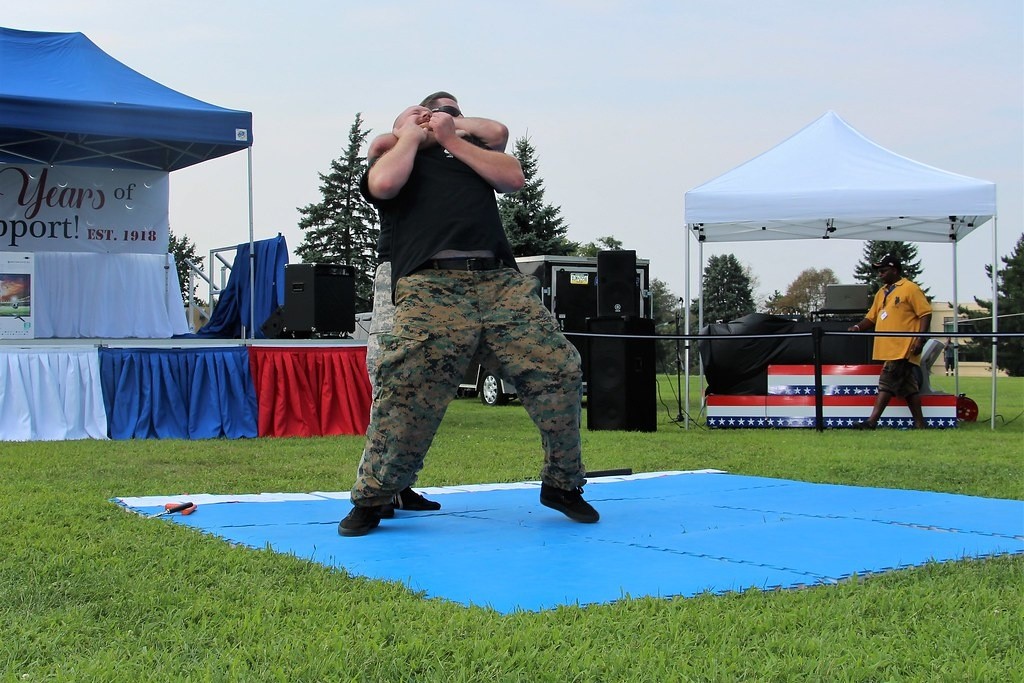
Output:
[430,105,464,117]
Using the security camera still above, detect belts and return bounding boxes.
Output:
[415,258,501,270]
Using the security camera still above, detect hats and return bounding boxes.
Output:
[872,253,902,270]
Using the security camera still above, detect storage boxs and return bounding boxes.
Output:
[826,283,868,309]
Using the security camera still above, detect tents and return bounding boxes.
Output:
[0,29,254,339]
[682,110,999,430]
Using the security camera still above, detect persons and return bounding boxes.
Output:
[847,253,932,429]
[944,337,955,377]
[338,106,600,538]
[366,91,510,517]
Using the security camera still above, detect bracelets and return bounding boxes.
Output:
[918,336,925,343]
[854,324,861,332]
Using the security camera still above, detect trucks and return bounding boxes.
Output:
[455,255,650,407]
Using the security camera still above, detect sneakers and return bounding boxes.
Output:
[380,503,395,518]
[540,481,600,524]
[338,502,380,537]
[390,486,441,510]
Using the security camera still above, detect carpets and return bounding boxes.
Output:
[105,468,1024,615]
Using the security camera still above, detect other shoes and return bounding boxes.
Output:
[951,373,954,376]
[907,421,927,430]
[946,372,948,376]
[852,420,878,430]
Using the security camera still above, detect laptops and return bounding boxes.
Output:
[818,284,868,312]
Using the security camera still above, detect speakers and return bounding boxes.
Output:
[260,304,313,339]
[596,250,639,317]
[283,262,356,334]
[585,316,657,434]
